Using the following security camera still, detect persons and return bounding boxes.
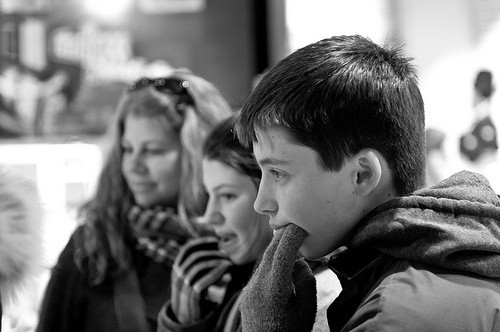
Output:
[34,67,235,332]
[232,34,500,332]
[157,111,349,332]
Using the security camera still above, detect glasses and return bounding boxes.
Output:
[126,77,195,107]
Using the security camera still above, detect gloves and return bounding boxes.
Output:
[170,236,233,324]
[239,225,317,331]
